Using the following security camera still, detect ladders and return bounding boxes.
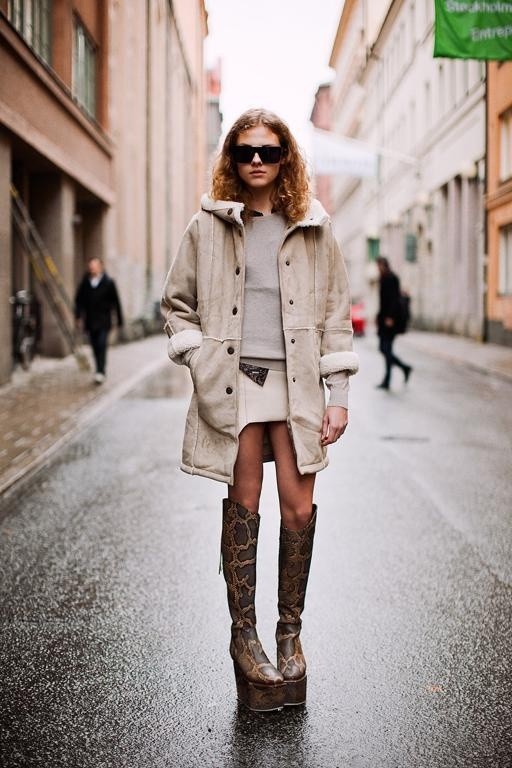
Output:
[10,184,94,377]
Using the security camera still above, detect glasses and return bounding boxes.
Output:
[230,146,283,164]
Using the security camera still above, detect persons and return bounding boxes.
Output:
[73,257,123,385]
[376,256,411,389]
[160,108,360,712]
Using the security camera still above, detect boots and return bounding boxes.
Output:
[275,504,317,706]
[221,498,287,712]
